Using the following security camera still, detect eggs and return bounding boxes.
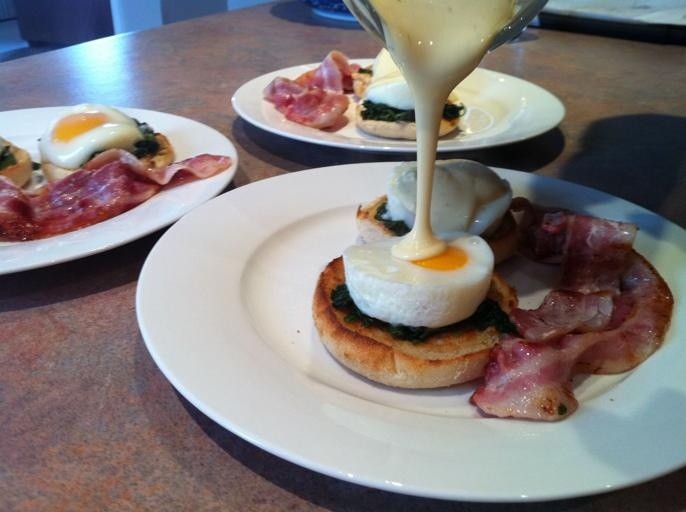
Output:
[342,229,496,330]
[37,104,144,171]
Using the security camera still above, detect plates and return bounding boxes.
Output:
[1,102,241,276]
[132,157,685,502]
[228,52,568,154]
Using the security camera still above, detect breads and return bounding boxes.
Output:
[41,133,174,185]
[311,256,519,390]
[355,104,460,140]
[1,137,32,188]
